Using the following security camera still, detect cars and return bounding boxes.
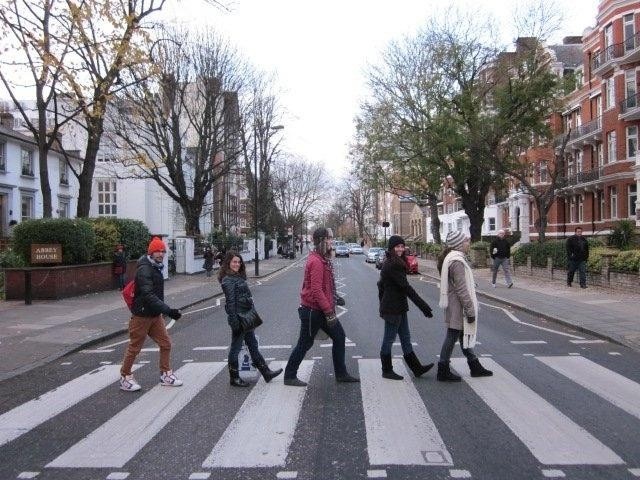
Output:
[349,243,364,254]
[367,248,386,262]
[402,248,419,274]
[376,251,390,268]
[336,246,350,258]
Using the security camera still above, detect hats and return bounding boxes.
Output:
[148,236,166,254]
[387,235,405,251]
[312,227,333,245]
[116,244,124,249]
[446,231,467,251]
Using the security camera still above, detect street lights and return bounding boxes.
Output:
[254,124,286,277]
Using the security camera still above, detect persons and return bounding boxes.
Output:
[119,237,183,391]
[437,230,493,381]
[114,244,127,291]
[490,230,513,289]
[565,226,589,289]
[283,228,360,386]
[376,235,434,380]
[203,246,214,277]
[360,239,365,248]
[217,250,283,387]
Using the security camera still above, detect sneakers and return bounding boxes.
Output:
[159,370,184,387]
[580,284,587,289]
[118,374,144,391]
[506,283,514,289]
[491,283,496,288]
[566,282,572,288]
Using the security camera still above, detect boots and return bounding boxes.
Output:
[226,361,251,387]
[283,369,310,387]
[379,353,405,380]
[466,357,494,378]
[254,357,283,384]
[403,350,433,378]
[335,367,361,383]
[436,360,462,382]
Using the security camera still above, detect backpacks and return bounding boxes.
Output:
[120,279,140,310]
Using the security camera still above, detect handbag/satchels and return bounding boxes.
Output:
[202,260,208,269]
[114,266,123,274]
[236,308,263,332]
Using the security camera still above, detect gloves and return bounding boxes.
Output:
[324,310,337,327]
[467,315,475,324]
[169,307,182,321]
[421,305,434,319]
[335,296,345,307]
